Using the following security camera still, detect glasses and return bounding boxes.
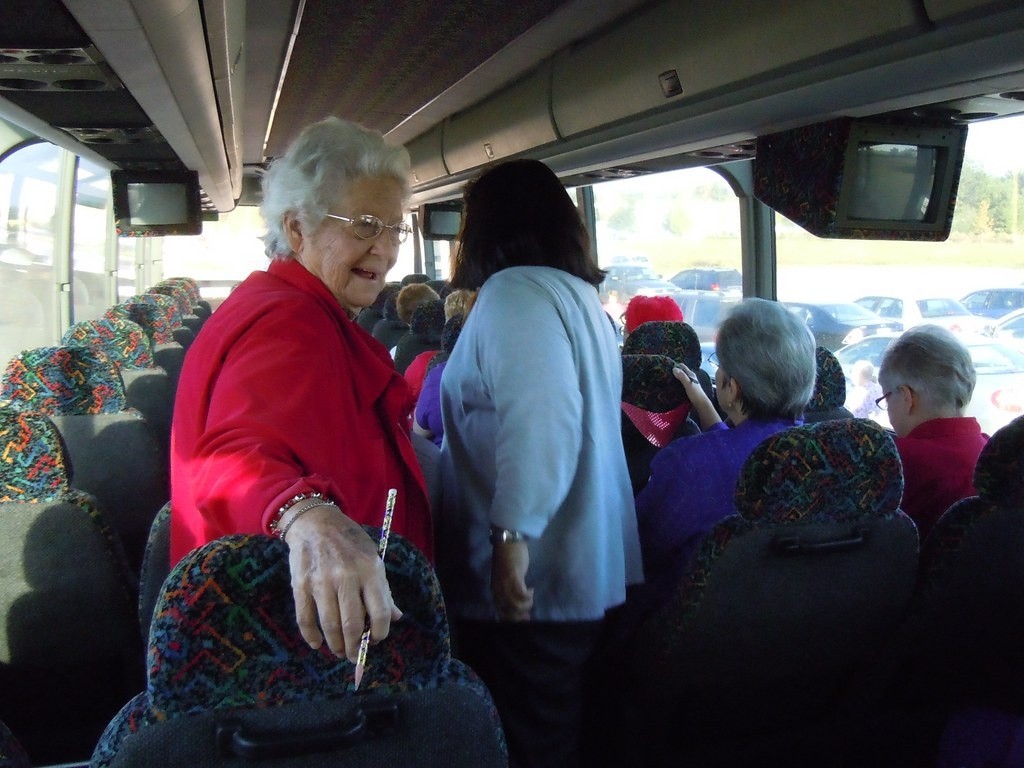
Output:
[324,211,415,245]
[708,351,743,396]
[875,385,913,411]
[619,326,630,338]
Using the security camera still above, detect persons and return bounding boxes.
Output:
[401,290,473,429]
[636,298,819,621]
[619,295,683,348]
[387,283,441,362]
[876,327,987,541]
[441,158,646,768]
[169,117,438,664]
[413,360,448,449]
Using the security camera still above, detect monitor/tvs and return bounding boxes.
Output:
[753,115,970,243]
[419,203,465,241]
[109,168,202,238]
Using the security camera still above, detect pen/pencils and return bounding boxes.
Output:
[688,377,699,384]
[354,489,398,691]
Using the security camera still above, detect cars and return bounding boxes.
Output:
[599,265,1021,441]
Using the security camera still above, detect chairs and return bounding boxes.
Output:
[0,274,1024,768]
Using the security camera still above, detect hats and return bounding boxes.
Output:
[625,295,684,332]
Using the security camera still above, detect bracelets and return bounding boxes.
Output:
[488,528,529,544]
[270,493,340,541]
